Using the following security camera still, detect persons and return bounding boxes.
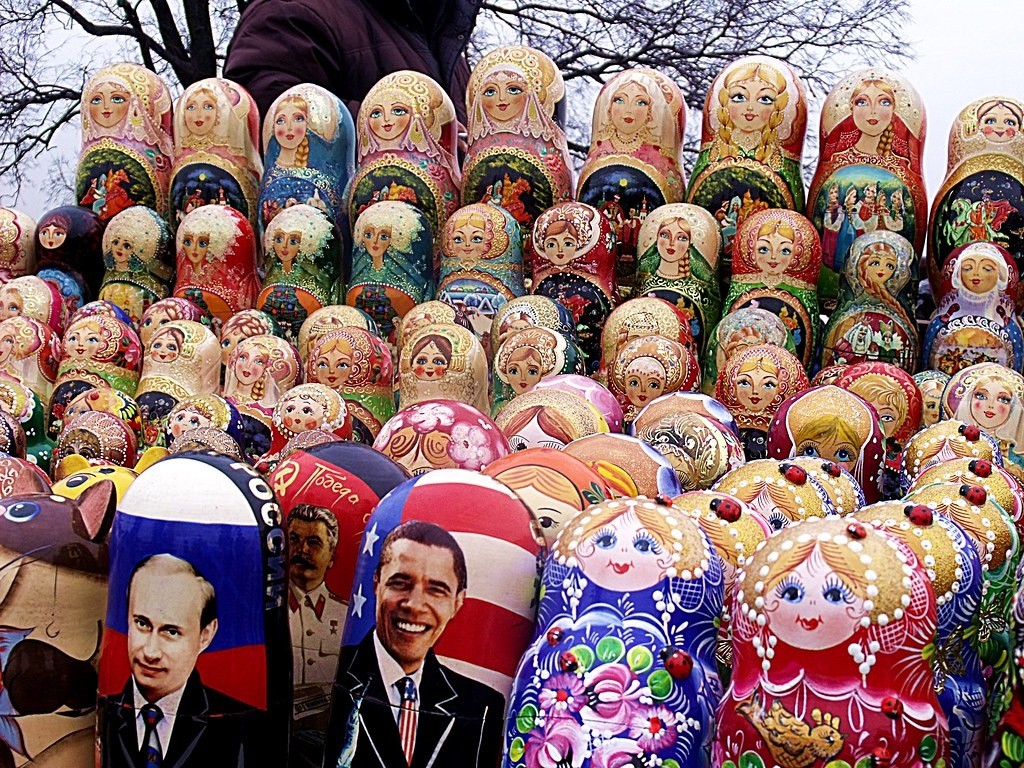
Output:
[323,520,504,768]
[98,554,268,768]
